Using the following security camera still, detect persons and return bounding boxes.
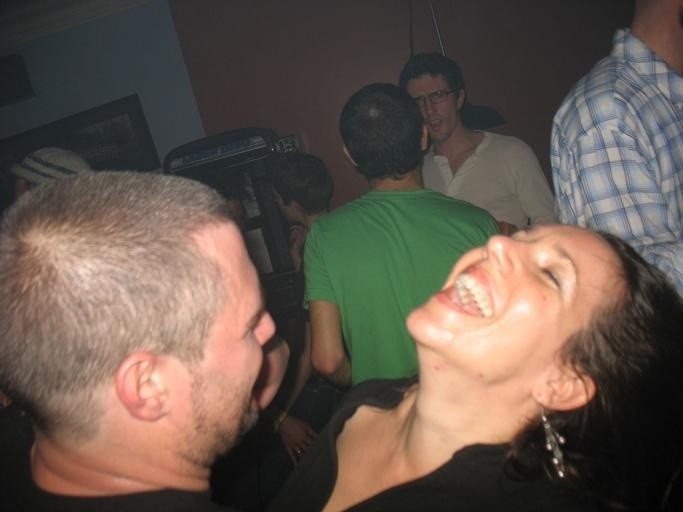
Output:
[548,0,682,296]
[1,136,333,469]
[253,221,683,512]
[0,170,277,512]
[300,81,505,389]
[397,52,553,232]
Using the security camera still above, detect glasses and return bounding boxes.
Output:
[412,89,456,107]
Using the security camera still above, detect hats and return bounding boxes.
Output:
[12,147,92,184]
